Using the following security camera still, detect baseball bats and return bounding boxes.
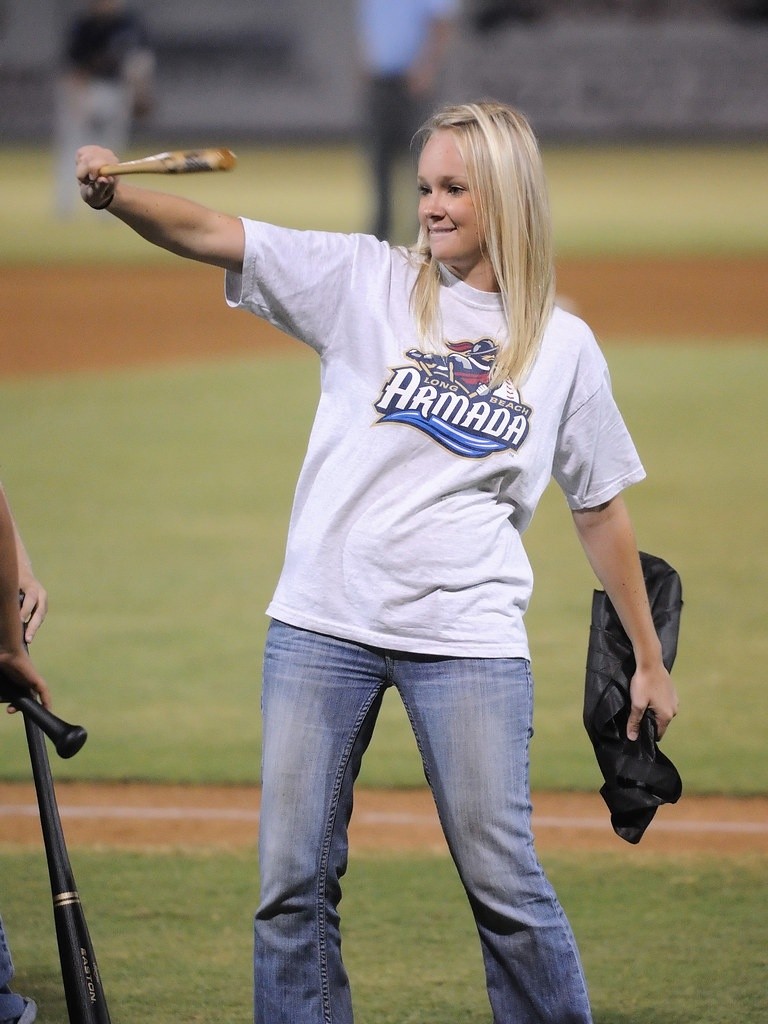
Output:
[3,675,88,761]
[13,683,108,1023]
[97,143,244,175]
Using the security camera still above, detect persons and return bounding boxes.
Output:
[342,1,463,242]
[48,1,155,227]
[76,103,679,1024]
[0,486,53,1024]
